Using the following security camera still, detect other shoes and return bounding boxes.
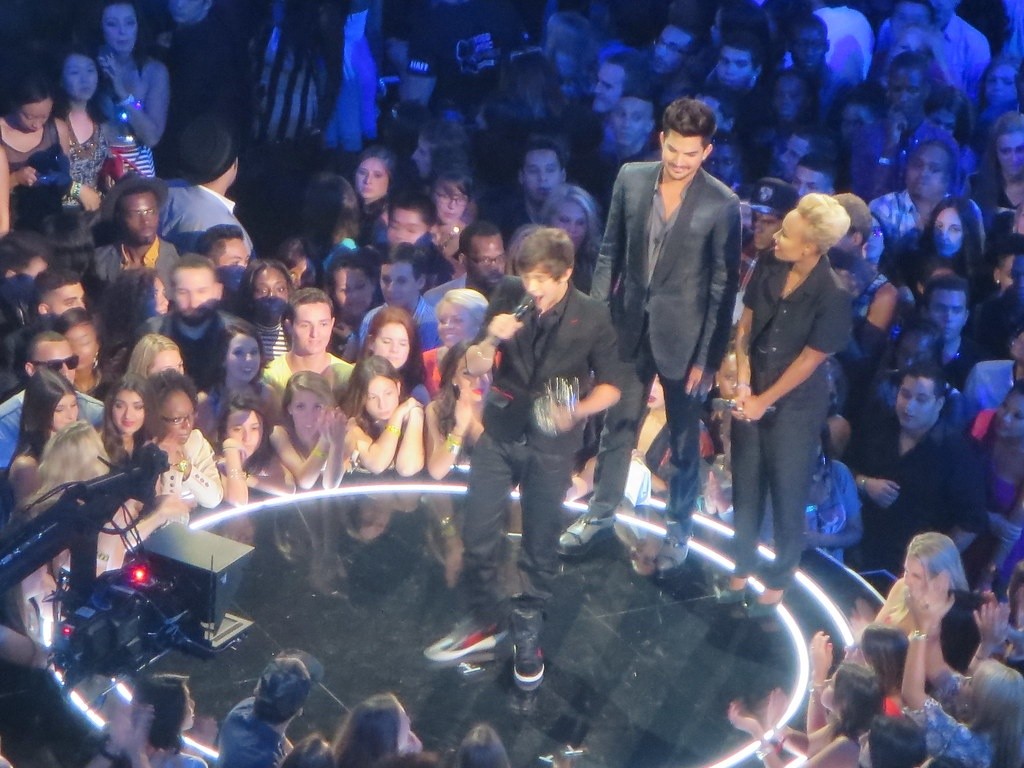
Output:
[557,512,616,556]
[718,575,749,604]
[747,589,789,618]
[654,519,696,580]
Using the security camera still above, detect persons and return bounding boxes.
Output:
[0,0,1024,768]
[421,233,624,682]
[556,98,742,580]
[716,192,852,617]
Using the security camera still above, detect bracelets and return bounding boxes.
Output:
[861,477,869,490]
[245,472,251,480]
[100,747,116,760]
[221,446,239,450]
[311,448,328,459]
[974,654,982,660]
[384,425,401,437]
[808,684,824,702]
[97,550,110,561]
[756,745,773,760]
[174,460,188,471]
[738,384,752,390]
[476,348,494,361]
[913,630,929,641]
[445,433,462,455]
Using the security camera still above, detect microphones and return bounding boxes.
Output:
[711,398,777,413]
[512,295,536,319]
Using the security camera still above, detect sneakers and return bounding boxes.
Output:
[423,609,509,661]
[509,608,544,683]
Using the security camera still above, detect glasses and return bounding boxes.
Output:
[29,354,79,371]
[161,412,198,425]
[433,188,469,206]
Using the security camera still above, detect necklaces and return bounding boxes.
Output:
[65,114,99,160]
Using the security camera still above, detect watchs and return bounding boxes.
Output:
[115,94,134,107]
[73,182,81,197]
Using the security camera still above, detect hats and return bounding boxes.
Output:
[100,171,168,228]
[740,176,798,219]
[170,117,236,185]
